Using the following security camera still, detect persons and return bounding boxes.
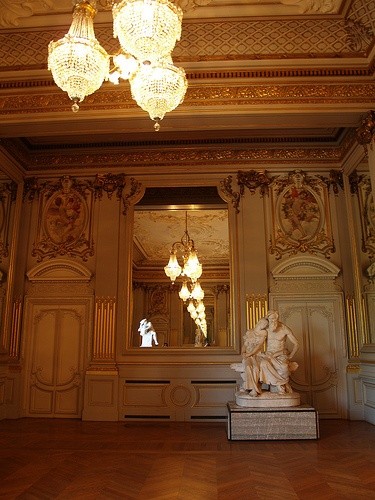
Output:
[260,312,301,395]
[243,318,270,398]
[137,318,158,346]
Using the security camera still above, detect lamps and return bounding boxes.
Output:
[44,0,188,134]
[163,211,210,340]
